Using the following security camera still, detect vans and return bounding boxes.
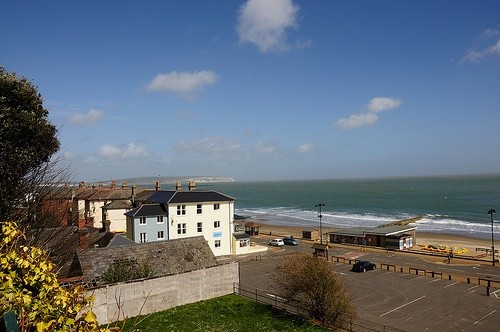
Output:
[268,238,284,246]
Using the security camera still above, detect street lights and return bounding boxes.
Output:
[488,207,497,266]
[315,203,326,244]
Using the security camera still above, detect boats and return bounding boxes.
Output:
[419,244,468,254]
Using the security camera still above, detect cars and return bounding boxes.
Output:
[353,260,376,273]
[283,237,299,245]
